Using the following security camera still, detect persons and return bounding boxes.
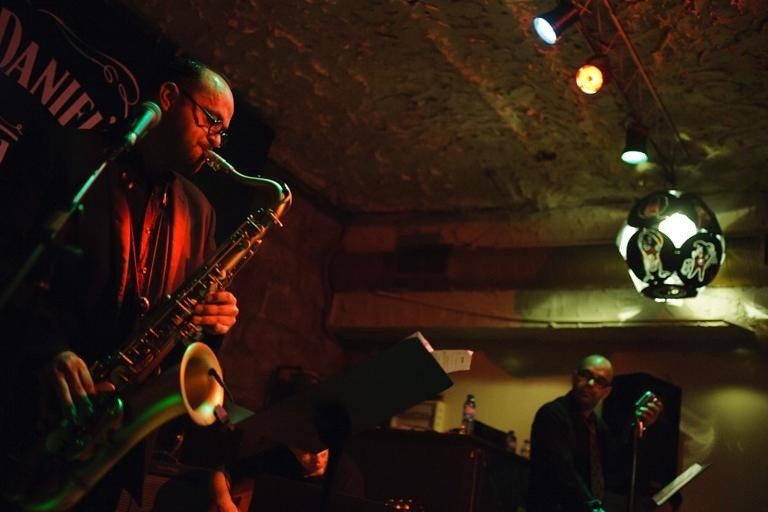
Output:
[526,353,663,511]
[1,56,240,510]
[209,447,328,511]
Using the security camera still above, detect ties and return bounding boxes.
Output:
[588,412,604,501]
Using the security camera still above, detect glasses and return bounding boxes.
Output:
[576,366,612,389]
[182,90,231,146]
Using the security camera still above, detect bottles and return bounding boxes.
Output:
[519,439,530,456]
[507,431,517,453]
[458,393,478,437]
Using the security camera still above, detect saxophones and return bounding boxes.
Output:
[0,149,292,512]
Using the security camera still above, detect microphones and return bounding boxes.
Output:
[635,390,654,410]
[123,101,161,151]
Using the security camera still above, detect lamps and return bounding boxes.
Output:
[616,190,728,299]
[531,0,693,171]
